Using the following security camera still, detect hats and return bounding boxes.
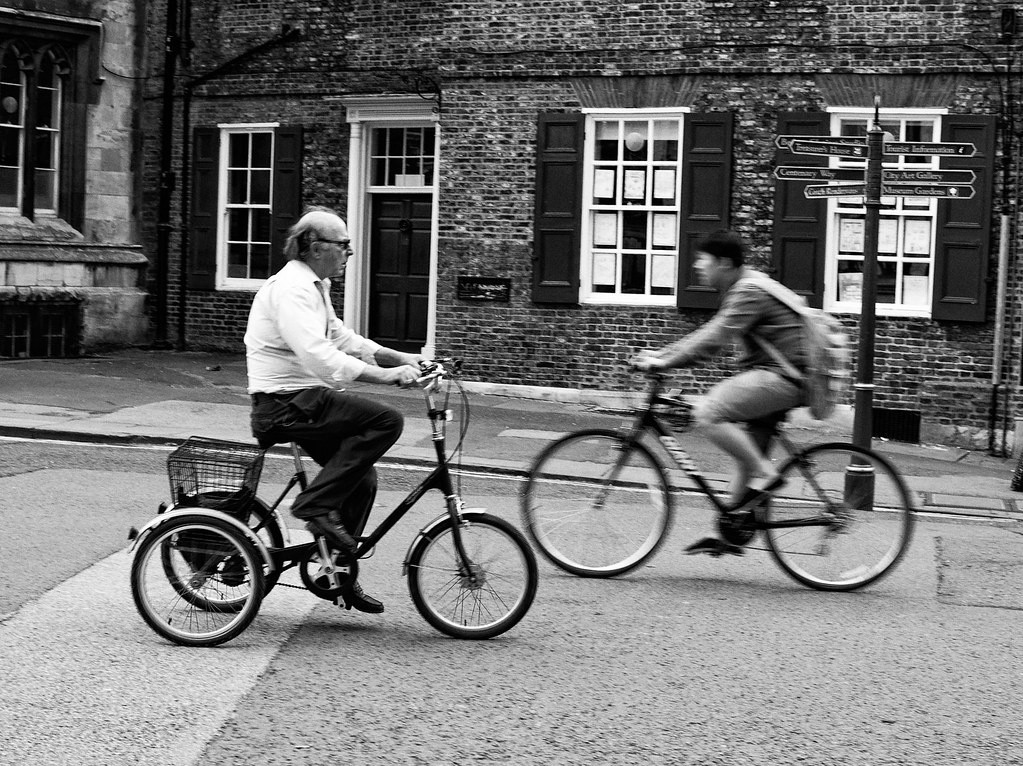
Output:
[692,229,747,259]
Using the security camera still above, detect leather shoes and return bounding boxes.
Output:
[307,510,357,549]
[314,566,384,613]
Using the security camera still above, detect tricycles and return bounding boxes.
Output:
[127,355,537,650]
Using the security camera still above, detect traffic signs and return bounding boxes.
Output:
[789,139,870,159]
[774,165,867,182]
[881,168,978,185]
[882,141,978,158]
[880,183,976,200]
[803,183,867,199]
[775,134,867,150]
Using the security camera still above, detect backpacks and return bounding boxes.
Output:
[730,277,851,421]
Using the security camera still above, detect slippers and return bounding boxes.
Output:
[724,476,787,514]
[681,536,747,558]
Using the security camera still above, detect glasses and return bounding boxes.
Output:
[315,237,351,249]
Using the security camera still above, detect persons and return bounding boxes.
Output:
[243,203,438,613]
[630,235,822,556]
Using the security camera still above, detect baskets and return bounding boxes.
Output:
[167,435,265,519]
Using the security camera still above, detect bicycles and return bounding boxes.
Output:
[522,362,915,596]
